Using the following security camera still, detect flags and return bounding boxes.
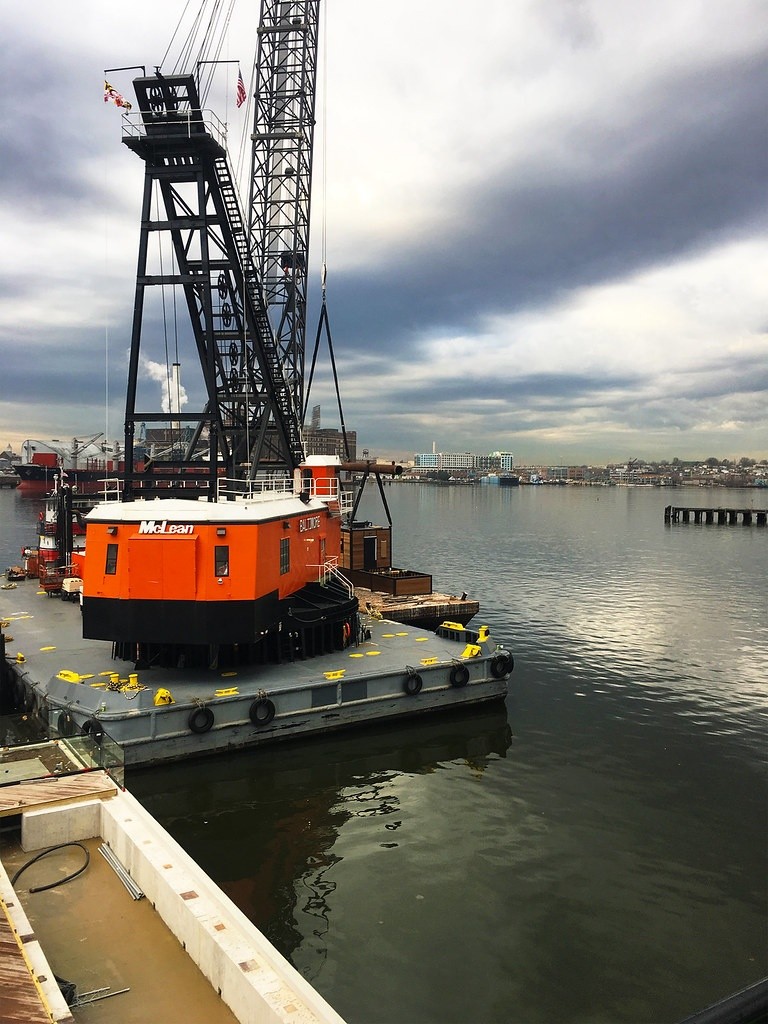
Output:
[104,80,132,111]
[236,69,246,108]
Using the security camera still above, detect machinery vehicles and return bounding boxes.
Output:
[79,0,361,661]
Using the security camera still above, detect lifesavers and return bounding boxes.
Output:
[346,623,351,637]
[403,673,422,695]
[249,698,275,726]
[39,512,44,520]
[490,655,510,678]
[7,668,73,738]
[188,707,214,733]
[508,651,514,674]
[81,719,103,748]
[21,547,24,555]
[449,666,469,687]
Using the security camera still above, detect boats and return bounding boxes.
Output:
[340,526,480,631]
[12,432,232,493]
